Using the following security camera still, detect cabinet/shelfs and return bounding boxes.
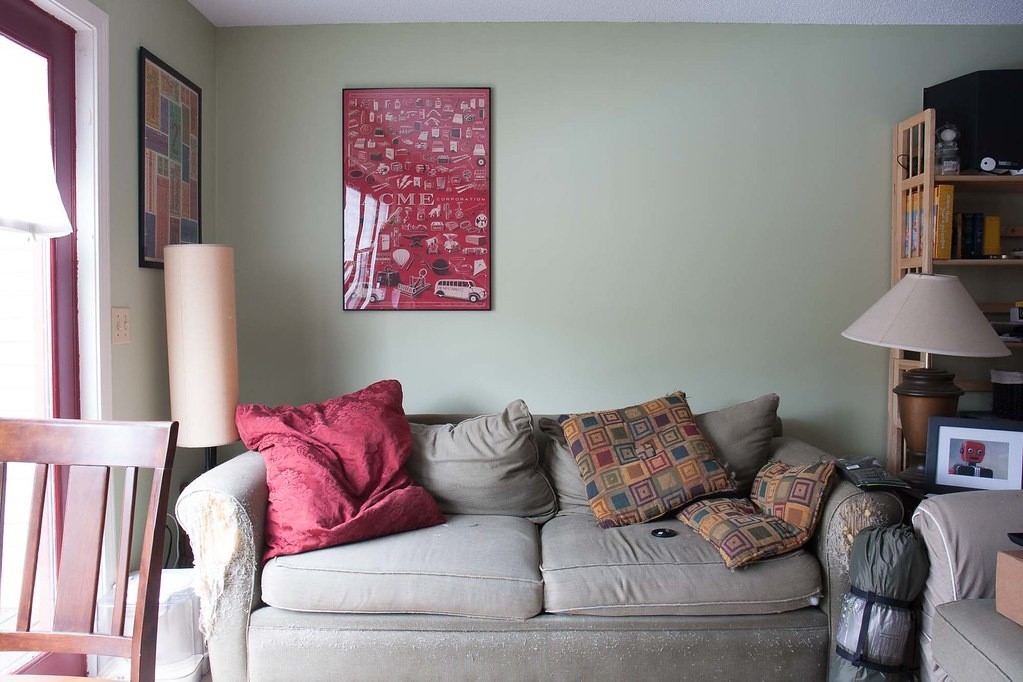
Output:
[887,108,1023,476]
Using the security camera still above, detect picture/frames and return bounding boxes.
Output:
[137,45,204,269]
[923,416,1023,496]
[341,87,492,312]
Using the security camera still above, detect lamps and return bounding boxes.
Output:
[840,272,1013,486]
[162,244,240,476]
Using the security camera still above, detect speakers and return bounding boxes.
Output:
[923,68,1022,179]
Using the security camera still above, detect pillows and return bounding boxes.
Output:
[673,458,836,571]
[538,392,781,516]
[556,390,742,531]
[407,398,559,525]
[234,379,450,566]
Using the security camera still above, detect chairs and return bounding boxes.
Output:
[0,415,178,681]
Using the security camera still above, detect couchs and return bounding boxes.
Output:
[913,490,1022,682]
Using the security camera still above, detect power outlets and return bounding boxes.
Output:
[111,307,130,345]
